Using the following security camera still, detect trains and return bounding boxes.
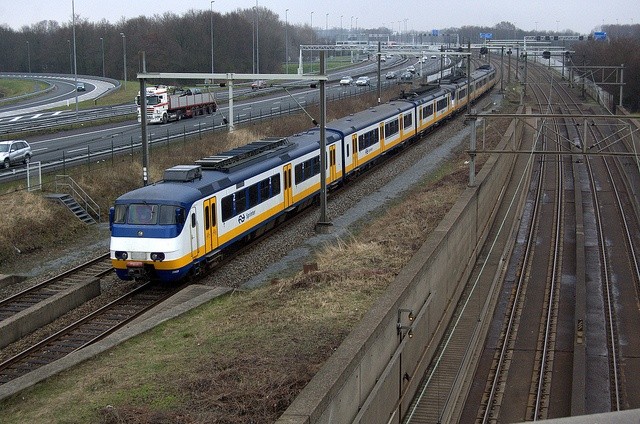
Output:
[109,64,497,284]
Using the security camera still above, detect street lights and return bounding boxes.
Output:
[398,308,414,424]
[120,32,127,92]
[339,14,344,35]
[25,40,31,74]
[100,36,105,78]
[67,40,73,76]
[390,18,410,34]
[209,0,216,84]
[355,17,358,30]
[285,8,290,75]
[251,5,257,74]
[310,11,315,71]
[325,12,330,44]
[351,15,354,30]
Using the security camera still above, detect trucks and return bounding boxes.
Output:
[135,84,216,124]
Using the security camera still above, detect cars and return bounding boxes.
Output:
[77,83,85,91]
[340,76,353,85]
[380,54,439,63]
[251,80,272,89]
[407,65,415,74]
[386,72,397,79]
[401,72,413,80]
[356,77,370,86]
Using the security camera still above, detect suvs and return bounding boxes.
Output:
[0,140,32,169]
